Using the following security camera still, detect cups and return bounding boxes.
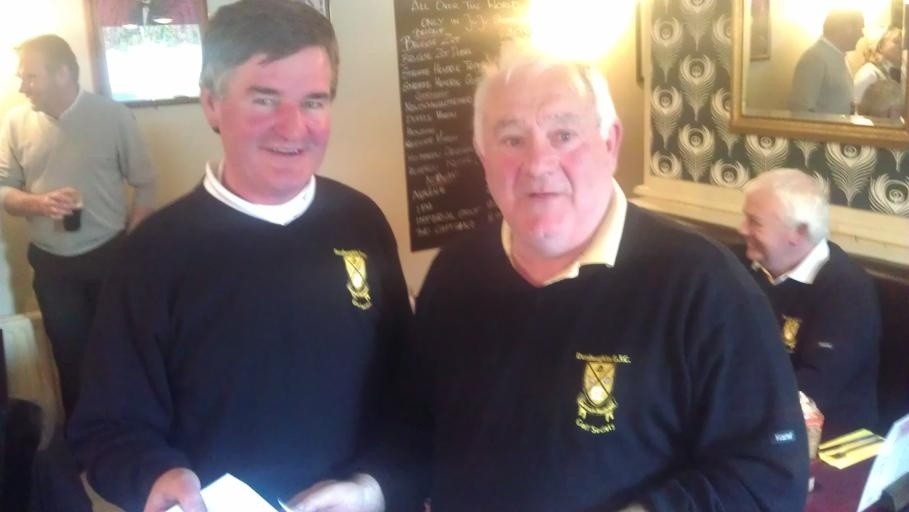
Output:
[804,416,825,460]
[64,201,84,231]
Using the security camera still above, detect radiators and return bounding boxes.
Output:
[0,309,84,478]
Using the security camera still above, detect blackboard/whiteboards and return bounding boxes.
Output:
[393,0,532,253]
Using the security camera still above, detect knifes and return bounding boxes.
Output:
[819,434,876,452]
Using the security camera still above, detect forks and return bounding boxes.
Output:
[830,439,882,459]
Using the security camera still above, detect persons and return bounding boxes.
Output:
[279,40,809,512]
[859,80,901,117]
[855,26,902,117]
[0,33,161,418]
[728,167,887,444]
[789,8,866,115]
[64,1,416,512]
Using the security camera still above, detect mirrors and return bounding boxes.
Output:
[729,0,909,148]
